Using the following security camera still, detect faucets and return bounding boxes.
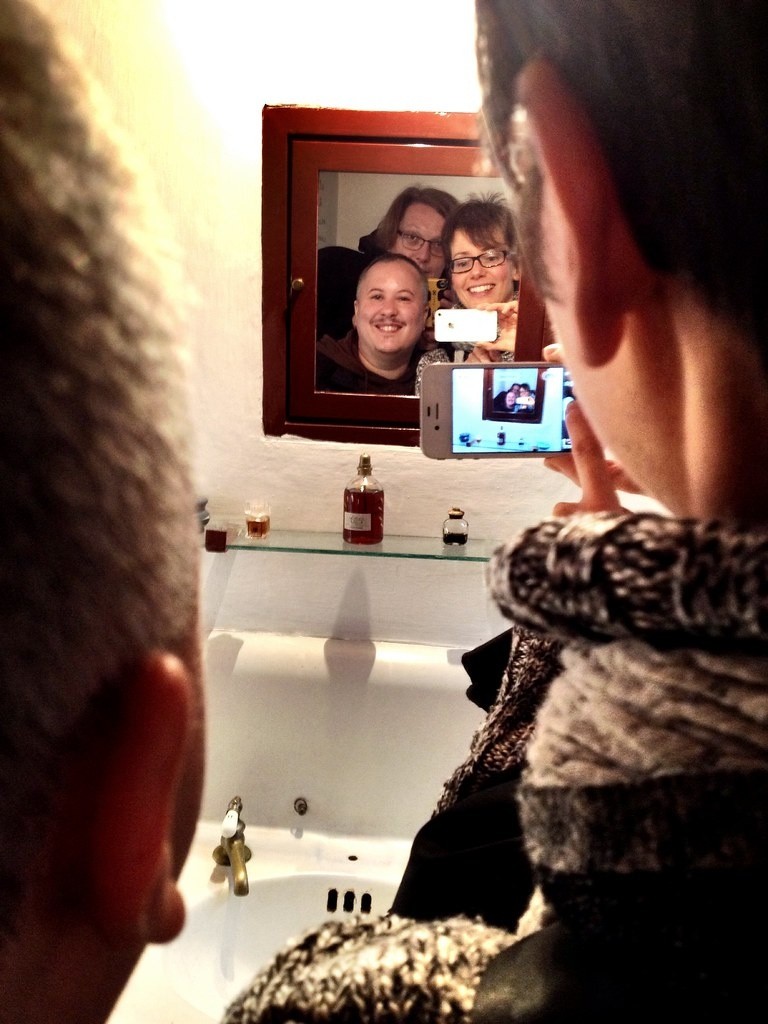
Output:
[211,796,251,898]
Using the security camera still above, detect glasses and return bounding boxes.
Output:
[395,229,445,258]
[449,250,510,273]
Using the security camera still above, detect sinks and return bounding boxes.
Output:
[155,872,392,1024]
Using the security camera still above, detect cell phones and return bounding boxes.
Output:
[434,309,498,342]
[420,362,579,461]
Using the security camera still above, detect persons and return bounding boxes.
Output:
[357,188,460,279]
[0,2,207,1024]
[414,200,519,397]
[494,383,536,412]
[315,254,431,396]
[392,1,768,1024]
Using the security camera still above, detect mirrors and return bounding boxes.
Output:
[482,369,545,424]
[287,140,543,423]
